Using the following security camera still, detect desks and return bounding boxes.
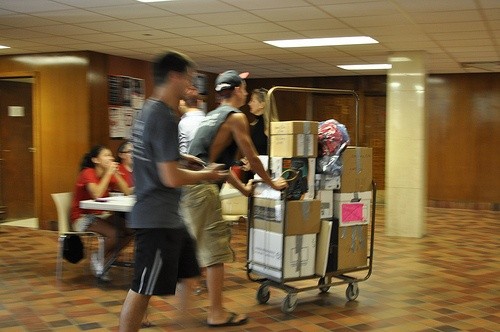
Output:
[79,188,241,279]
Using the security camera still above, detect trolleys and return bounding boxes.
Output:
[239,85,378,313]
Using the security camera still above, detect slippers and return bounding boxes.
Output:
[207,312,248,327]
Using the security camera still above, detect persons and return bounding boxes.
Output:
[117,50,218,332]
[238,87,279,185]
[116,139,138,252]
[176,85,208,156]
[68,145,131,281]
[177,68,289,329]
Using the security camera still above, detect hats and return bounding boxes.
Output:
[214,70,250,95]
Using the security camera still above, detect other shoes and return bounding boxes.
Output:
[100,270,111,281]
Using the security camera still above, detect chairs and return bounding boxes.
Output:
[219,195,249,262]
[51,193,107,282]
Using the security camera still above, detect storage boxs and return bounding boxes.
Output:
[249,121,373,283]
